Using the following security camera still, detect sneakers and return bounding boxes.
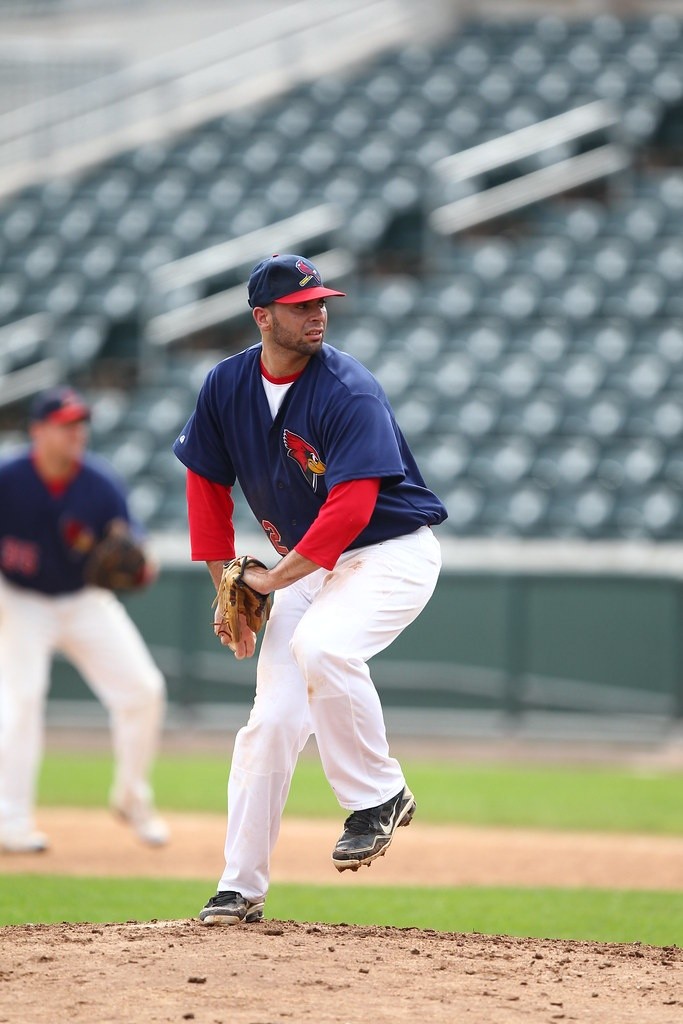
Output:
[331,784,417,873]
[1,817,49,856]
[106,801,173,849]
[199,888,266,928]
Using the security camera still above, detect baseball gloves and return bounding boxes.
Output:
[209,555,275,643]
[83,537,147,592]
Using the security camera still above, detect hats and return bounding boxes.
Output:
[27,383,93,428]
[247,251,348,307]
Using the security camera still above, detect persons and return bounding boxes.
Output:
[0,385,172,853]
[173,255,448,926]
